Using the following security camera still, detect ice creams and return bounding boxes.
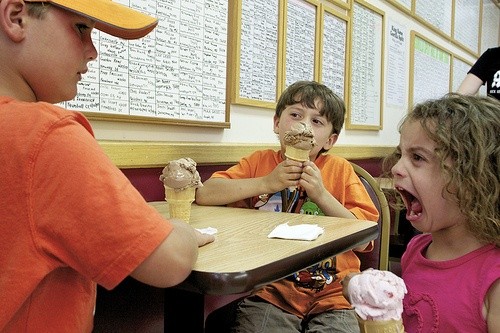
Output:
[284,121,317,168]
[159,157,203,223]
[347,268,408,333]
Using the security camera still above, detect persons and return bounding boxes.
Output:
[456,47,500,102]
[197,80,380,333]
[0,0,214,333]
[376,92,500,333]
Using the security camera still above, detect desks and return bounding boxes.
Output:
[148,197,381,333]
[373,176,399,234]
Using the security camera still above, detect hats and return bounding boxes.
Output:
[27,0,159,40]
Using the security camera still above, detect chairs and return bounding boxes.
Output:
[350,162,390,271]
